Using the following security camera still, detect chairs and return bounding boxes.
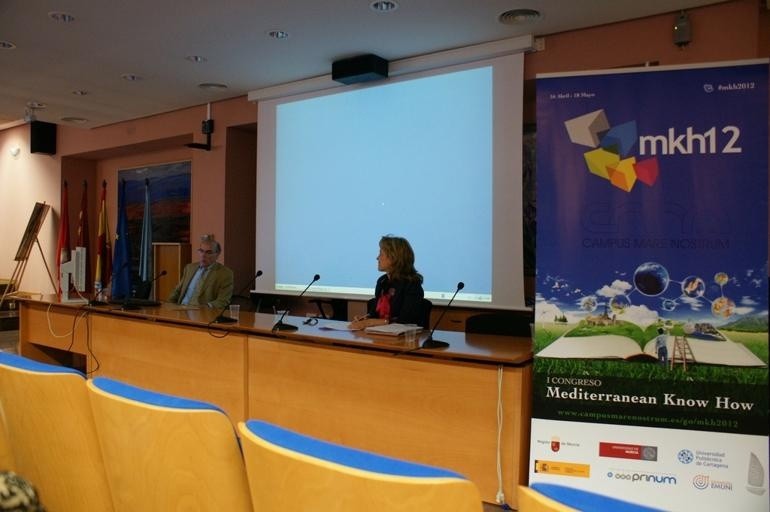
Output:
[237,419,484,510]
[85,377,250,511]
[0,350,114,512]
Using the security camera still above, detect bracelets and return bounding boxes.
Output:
[383,316,388,326]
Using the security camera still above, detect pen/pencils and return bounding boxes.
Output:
[352,313,370,323]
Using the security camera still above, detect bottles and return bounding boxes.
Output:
[94,278,102,298]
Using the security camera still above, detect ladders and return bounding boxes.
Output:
[670,335,697,372]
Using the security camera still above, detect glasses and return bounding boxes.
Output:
[197,249,217,255]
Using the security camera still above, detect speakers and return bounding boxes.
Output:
[30,120,56,155]
[332,54,388,85]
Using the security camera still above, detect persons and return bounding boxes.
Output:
[347,235,425,330]
[165,238,235,311]
[653,325,671,369]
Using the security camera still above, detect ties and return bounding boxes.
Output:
[196,267,207,281]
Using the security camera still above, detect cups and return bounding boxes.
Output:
[104,288,110,298]
[229,304,240,318]
[278,311,289,322]
[403,323,417,343]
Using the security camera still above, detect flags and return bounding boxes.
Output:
[138,176,156,297]
[112,178,134,303]
[94,179,113,301]
[75,179,92,297]
[54,180,72,302]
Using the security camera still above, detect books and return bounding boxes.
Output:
[364,322,425,338]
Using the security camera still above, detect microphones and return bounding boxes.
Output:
[272,274,320,331]
[120,270,166,310]
[88,262,128,305]
[214,270,262,323]
[422,282,464,348]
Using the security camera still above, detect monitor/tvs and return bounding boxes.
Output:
[59,247,88,304]
